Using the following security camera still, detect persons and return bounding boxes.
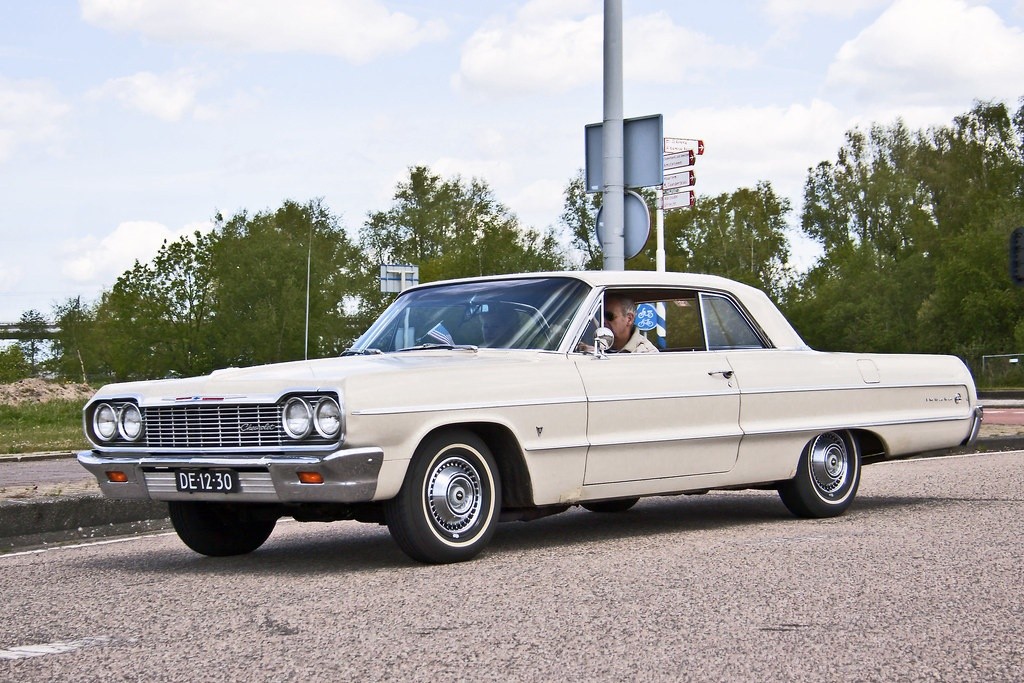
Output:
[478,304,521,348]
[575,294,659,354]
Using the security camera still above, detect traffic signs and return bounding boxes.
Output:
[662,137,705,209]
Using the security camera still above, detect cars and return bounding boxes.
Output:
[76,269,980,567]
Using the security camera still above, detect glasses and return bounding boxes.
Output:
[605,311,617,321]
